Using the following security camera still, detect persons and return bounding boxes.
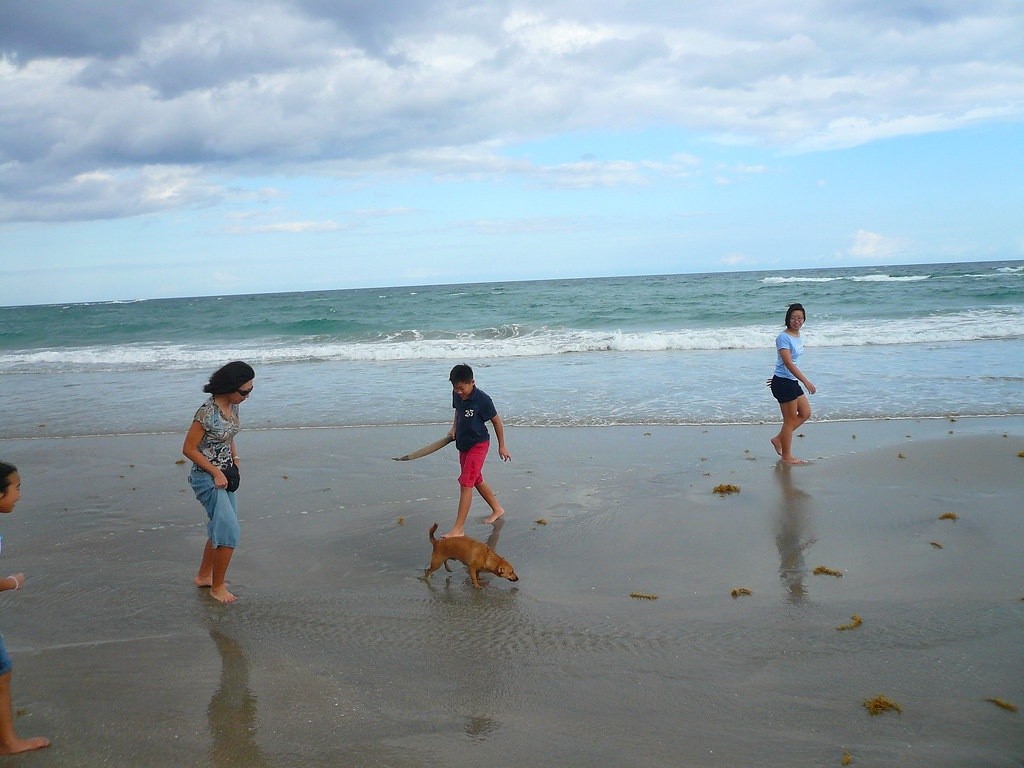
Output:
[183,362,256,603]
[0,459,52,755]
[766,302,816,464]
[440,363,511,539]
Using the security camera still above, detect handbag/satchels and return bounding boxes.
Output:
[221,464,241,493]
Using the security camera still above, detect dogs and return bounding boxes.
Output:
[422,523,519,590]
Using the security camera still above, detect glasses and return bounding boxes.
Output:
[236,386,255,396]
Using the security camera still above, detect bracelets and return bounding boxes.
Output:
[233,456,239,461]
[7,576,19,592]
